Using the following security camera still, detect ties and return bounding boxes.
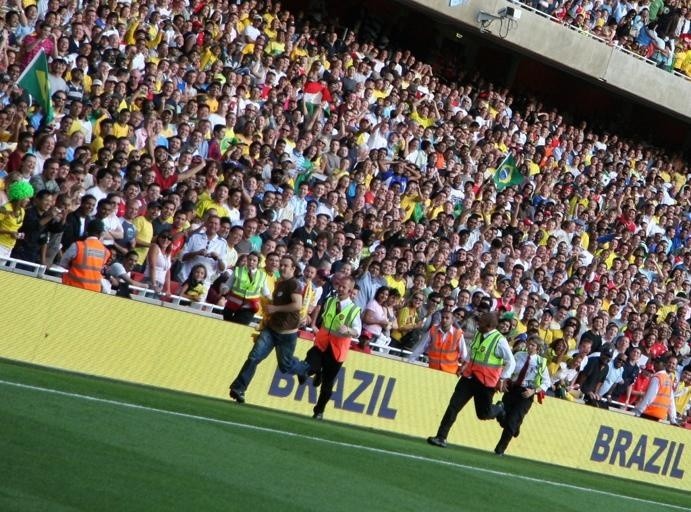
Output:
[515,355,532,385]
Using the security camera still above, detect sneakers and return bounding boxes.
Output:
[427,437,447,447]
[312,411,324,422]
[313,370,323,387]
[496,401,507,424]
[230,389,244,403]
